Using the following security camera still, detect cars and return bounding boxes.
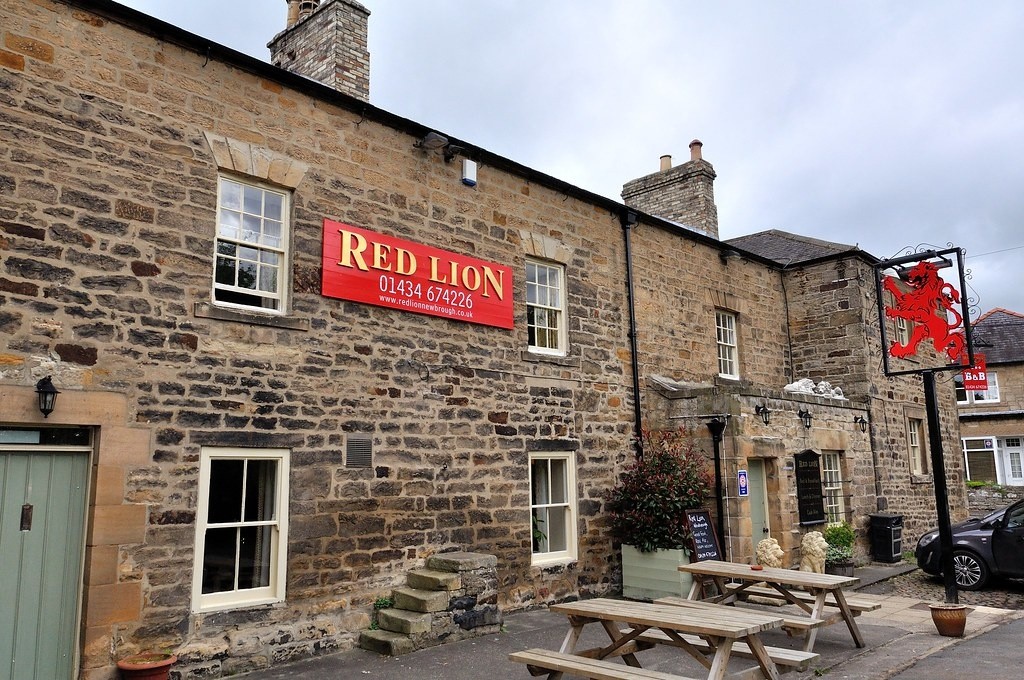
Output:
[914,498,1024,592]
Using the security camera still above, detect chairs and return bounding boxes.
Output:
[620,628,820,673]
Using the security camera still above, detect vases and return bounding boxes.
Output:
[928,604,967,637]
[116,652,178,680]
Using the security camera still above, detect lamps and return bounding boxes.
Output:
[755,403,771,426]
[444,144,464,163]
[854,414,867,433]
[412,132,449,150]
[799,409,813,430]
[34,374,62,418]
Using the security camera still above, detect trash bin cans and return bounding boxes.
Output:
[868,513,903,563]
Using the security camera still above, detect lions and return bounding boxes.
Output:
[755,538,785,588]
[800,531,829,590]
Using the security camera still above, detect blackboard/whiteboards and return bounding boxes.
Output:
[684,508,728,581]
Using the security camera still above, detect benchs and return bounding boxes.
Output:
[507,648,698,680]
[654,596,826,636]
[725,583,881,612]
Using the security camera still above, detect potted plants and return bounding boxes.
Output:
[532,515,547,553]
[601,424,713,603]
[821,506,859,586]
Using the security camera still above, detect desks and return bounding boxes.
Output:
[545,598,784,680]
[678,560,866,673]
